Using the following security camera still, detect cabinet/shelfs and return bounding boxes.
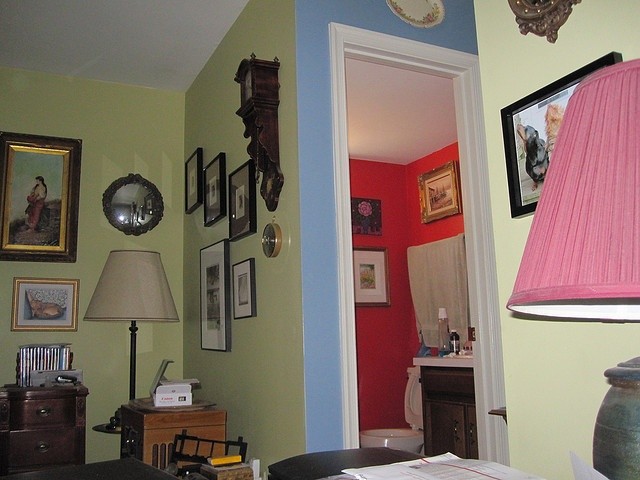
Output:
[120,400,231,472]
[422,366,477,458]
[0,385,88,475]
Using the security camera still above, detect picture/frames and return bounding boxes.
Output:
[228,159,257,242]
[203,153,227,227]
[0,132,82,262]
[418,161,462,225]
[233,258,257,320]
[200,238,233,353]
[185,147,202,214]
[500,52,622,218]
[387,1,445,29]
[351,198,383,237]
[353,247,390,307]
[10,277,80,332]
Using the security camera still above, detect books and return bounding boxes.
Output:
[51,381,81,387]
[21,348,70,386]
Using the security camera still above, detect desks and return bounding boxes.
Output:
[266,449,493,480]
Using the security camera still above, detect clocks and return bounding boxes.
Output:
[234,53,284,257]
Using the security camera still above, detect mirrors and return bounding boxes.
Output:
[102,172,165,236]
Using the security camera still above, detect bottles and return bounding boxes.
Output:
[437,307,450,357]
[449,329,460,355]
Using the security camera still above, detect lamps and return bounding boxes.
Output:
[506,61,640,480]
[84,250,179,426]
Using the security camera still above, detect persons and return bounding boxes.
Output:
[22,175,48,234]
[516,124,548,190]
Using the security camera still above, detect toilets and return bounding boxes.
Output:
[359,365,425,453]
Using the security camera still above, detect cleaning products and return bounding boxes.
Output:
[438,307,448,355]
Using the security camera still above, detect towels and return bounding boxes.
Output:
[407,233,468,350]
